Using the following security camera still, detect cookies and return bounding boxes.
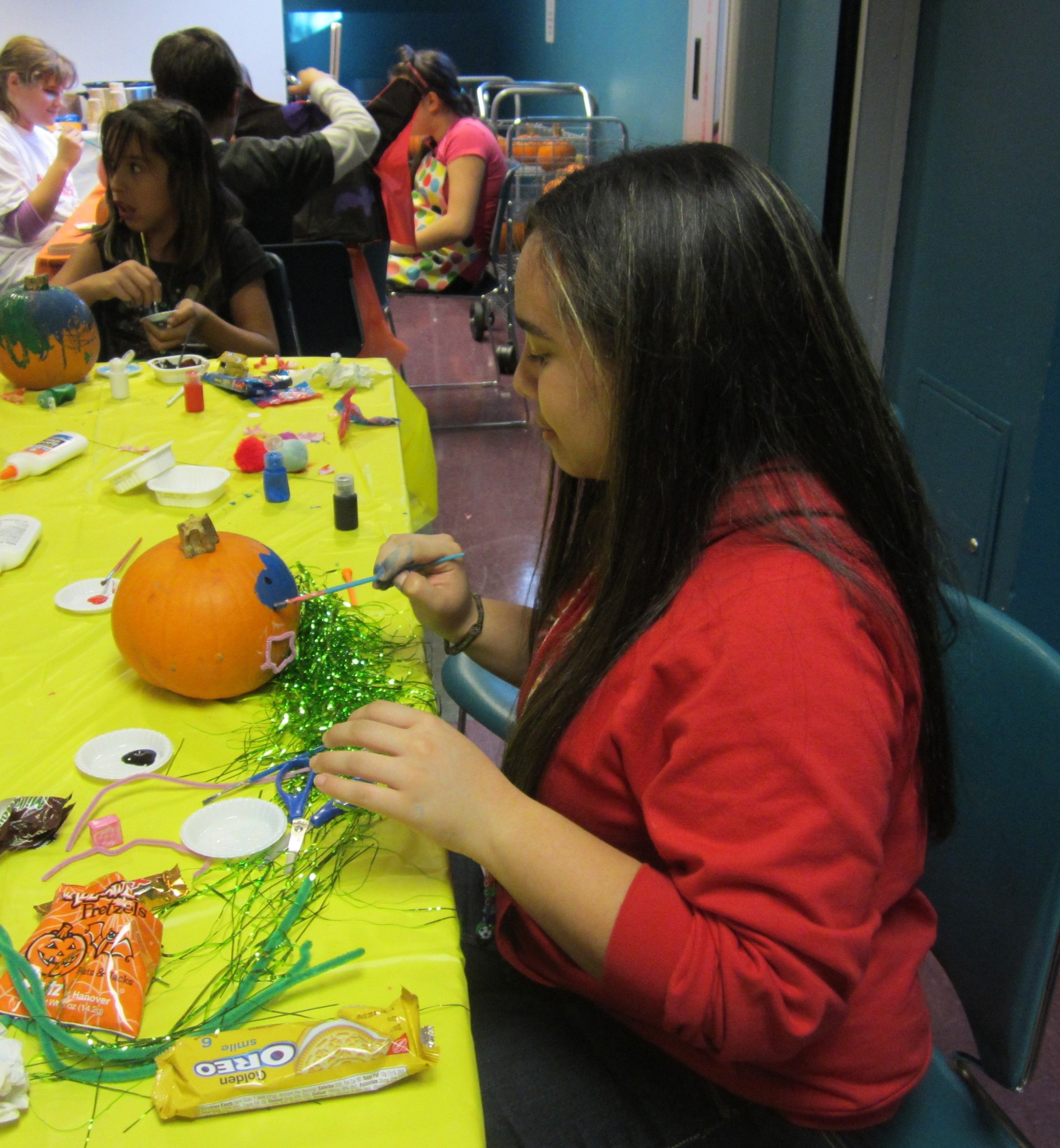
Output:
[293,1017,392,1074]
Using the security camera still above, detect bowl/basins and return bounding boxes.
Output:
[146,464,231,508]
[105,441,175,494]
[140,310,178,329]
[147,354,209,383]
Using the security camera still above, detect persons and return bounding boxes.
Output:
[151,26,380,255]
[385,50,506,296]
[309,141,966,1148]
[45,97,282,357]
[0,34,85,297]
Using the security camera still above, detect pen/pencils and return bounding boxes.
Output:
[82,137,102,149]
[165,385,184,409]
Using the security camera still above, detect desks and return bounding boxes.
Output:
[0,354,492,1147]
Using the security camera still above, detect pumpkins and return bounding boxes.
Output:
[493,121,587,193]
[496,216,526,255]
[111,513,301,698]
[0,273,101,391]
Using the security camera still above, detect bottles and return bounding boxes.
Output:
[0,432,88,481]
[184,371,204,412]
[109,349,134,399]
[333,474,358,531]
[264,452,290,503]
[37,383,76,408]
[0,514,41,573]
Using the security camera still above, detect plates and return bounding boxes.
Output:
[180,797,288,859]
[76,728,172,779]
[55,578,120,613]
[96,364,143,377]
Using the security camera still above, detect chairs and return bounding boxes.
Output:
[839,577,1060,1148]
[262,240,365,362]
[440,652,521,759]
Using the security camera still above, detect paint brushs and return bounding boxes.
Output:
[140,231,158,314]
[202,744,328,805]
[101,538,142,586]
[274,552,465,608]
[175,323,193,370]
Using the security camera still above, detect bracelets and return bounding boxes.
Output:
[443,592,484,656]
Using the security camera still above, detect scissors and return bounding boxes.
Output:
[258,757,374,874]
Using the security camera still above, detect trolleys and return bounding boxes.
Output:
[454,73,630,375]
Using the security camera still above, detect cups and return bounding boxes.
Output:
[109,82,126,111]
[86,88,106,133]
